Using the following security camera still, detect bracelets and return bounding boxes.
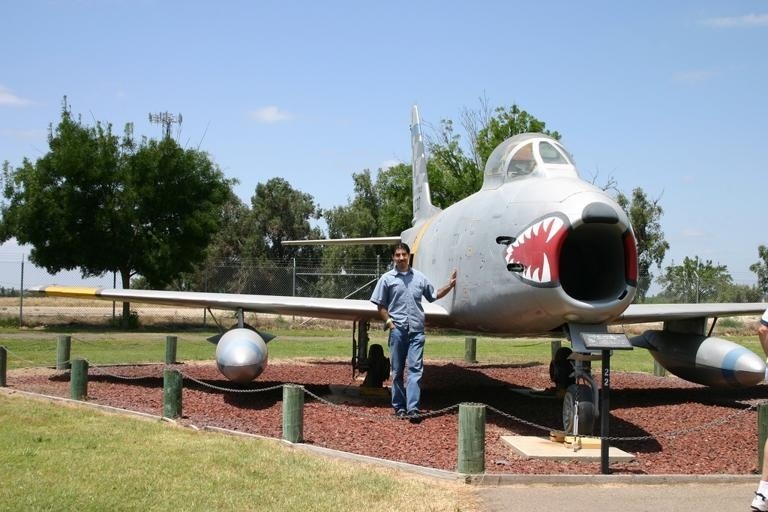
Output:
[385,319,392,326]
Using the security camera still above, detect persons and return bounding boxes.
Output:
[370,243,456,424]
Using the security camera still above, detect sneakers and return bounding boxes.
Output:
[396,408,421,421]
[750,491,768,512]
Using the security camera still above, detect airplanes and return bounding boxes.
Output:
[26,104,768,437]
